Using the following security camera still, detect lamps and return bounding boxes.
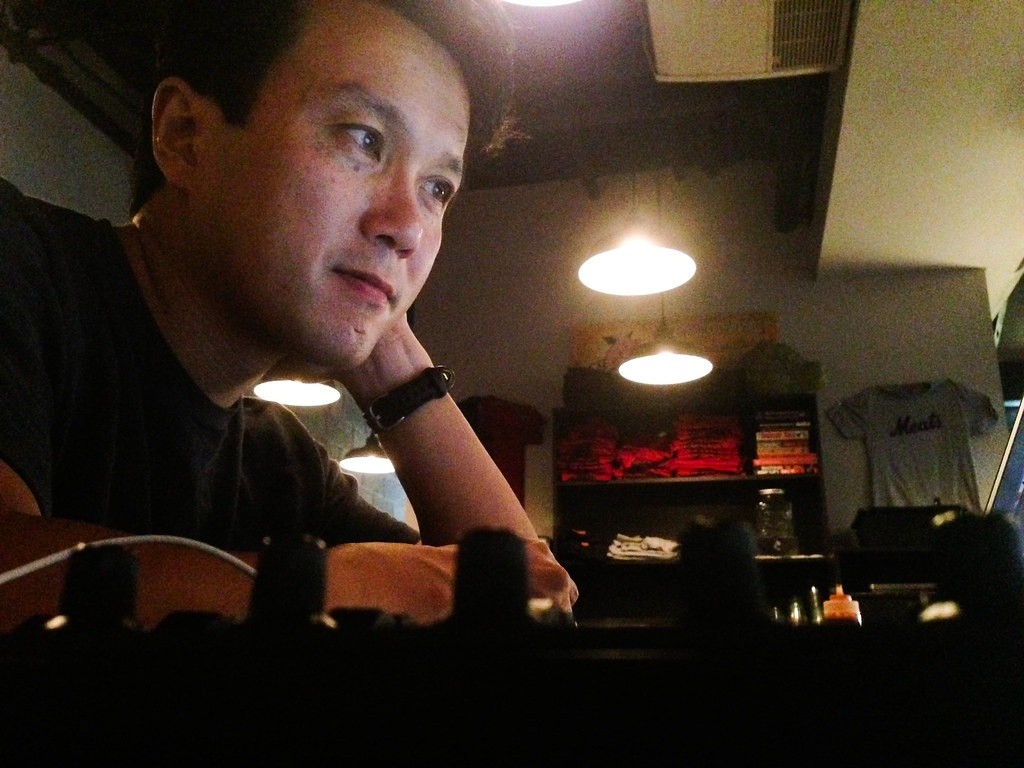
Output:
[575,154,714,386]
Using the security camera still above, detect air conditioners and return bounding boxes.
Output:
[640,0,846,82]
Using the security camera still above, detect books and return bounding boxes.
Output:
[752,410,818,475]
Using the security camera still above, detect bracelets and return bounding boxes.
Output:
[361,363,455,435]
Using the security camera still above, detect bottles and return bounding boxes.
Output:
[774,586,862,626]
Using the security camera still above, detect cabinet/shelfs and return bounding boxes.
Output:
[551,387,834,671]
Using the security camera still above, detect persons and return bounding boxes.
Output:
[0,0,583,635]
